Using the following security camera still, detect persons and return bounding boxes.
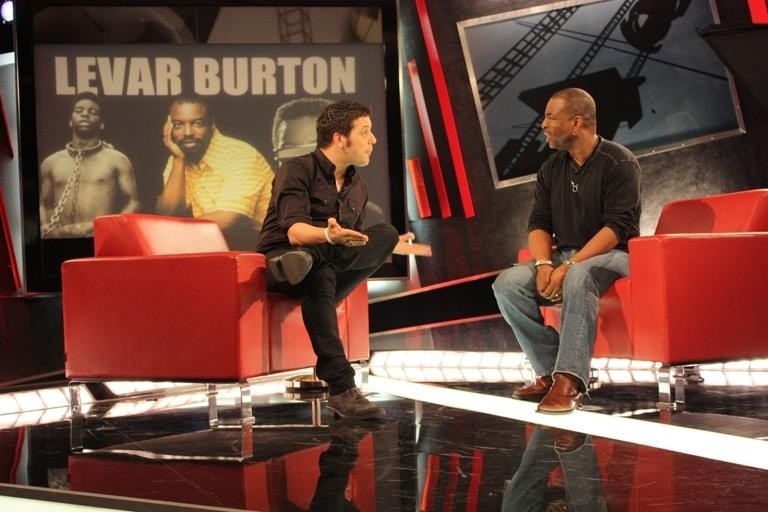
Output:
[38,91,140,238]
[500,422,607,511]
[270,98,335,165]
[264,425,363,511]
[491,87,642,412]
[257,101,433,423]
[152,95,276,250]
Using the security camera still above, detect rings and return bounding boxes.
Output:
[554,293,560,298]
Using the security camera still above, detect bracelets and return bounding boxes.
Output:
[534,259,557,267]
[323,226,336,245]
[563,260,576,268]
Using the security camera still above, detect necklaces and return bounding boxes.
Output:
[567,155,591,193]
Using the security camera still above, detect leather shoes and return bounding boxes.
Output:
[512,376,553,399]
[537,372,583,413]
[330,423,374,443]
[326,385,386,421]
[268,249,314,286]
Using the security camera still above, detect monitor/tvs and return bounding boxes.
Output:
[11,1,412,300]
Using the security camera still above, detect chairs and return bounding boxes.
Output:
[60,214,370,464]
[518,188,768,413]
